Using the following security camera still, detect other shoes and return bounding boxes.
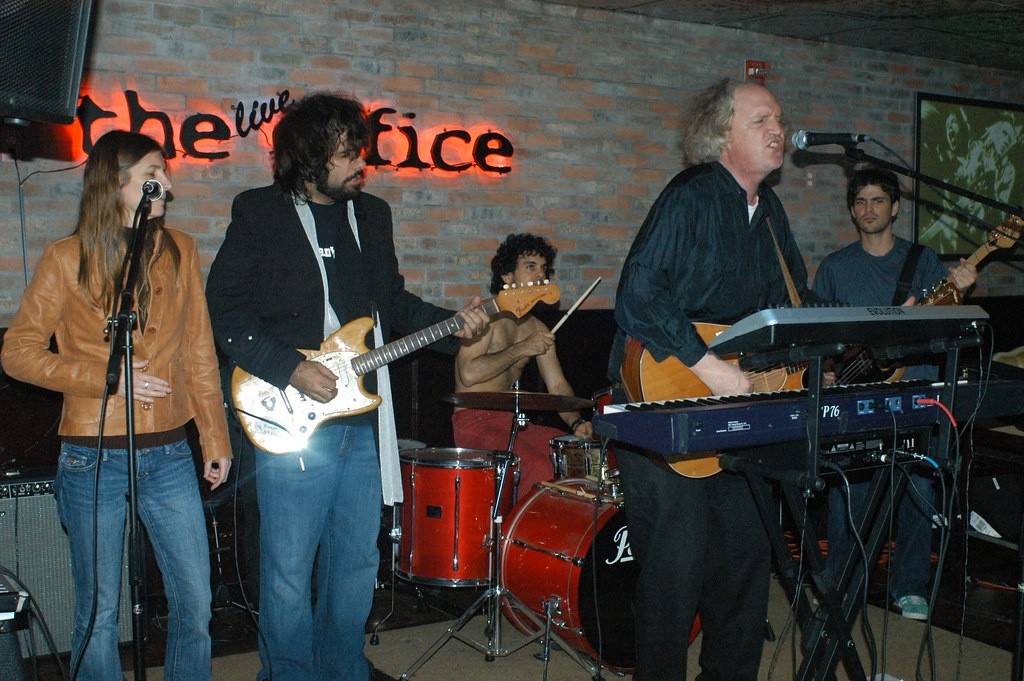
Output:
[370,669,397,681]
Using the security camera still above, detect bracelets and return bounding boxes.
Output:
[571,417,585,433]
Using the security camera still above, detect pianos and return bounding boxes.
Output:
[704,302,991,377]
[591,378,1024,681]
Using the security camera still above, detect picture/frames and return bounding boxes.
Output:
[912,91,1024,261]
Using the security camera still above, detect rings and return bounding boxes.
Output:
[143,381,148,389]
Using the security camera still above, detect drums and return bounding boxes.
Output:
[499,477,701,674]
[395,446,521,588]
[395,438,427,450]
[549,433,610,481]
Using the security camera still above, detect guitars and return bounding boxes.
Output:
[824,213,1024,385]
[621,277,963,479]
[231,280,561,456]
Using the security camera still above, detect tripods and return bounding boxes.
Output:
[399,395,607,681]
[205,504,260,618]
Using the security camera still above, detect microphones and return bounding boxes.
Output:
[792,129,872,151]
[141,179,163,201]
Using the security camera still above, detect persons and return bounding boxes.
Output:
[938,105,1024,256]
[0,129,232,681]
[455,235,593,504]
[811,163,977,619]
[609,78,836,681]
[205,90,490,680]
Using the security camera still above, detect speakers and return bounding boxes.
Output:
[0,468,149,661]
[0,0,94,127]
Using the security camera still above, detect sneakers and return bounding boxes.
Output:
[892,595,929,620]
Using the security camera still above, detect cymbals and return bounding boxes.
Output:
[442,391,597,412]
